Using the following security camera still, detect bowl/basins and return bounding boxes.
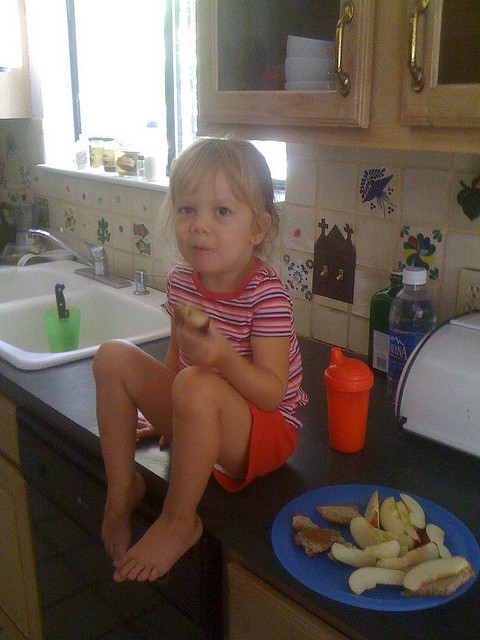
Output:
[283,34,338,89]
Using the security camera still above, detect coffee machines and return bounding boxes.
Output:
[0,193,48,259]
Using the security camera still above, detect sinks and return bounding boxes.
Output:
[0,258,145,307]
[0,283,174,371]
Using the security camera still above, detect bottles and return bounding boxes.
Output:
[136,156,146,178]
[101,138,115,172]
[387,267,438,405]
[73,132,88,171]
[115,141,142,176]
[88,137,101,168]
[324,346,375,453]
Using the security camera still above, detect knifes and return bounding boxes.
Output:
[54,282,71,320]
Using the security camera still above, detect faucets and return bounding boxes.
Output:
[27,228,108,275]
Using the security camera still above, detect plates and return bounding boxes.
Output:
[272,483,480,614]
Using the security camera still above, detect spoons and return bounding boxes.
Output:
[17,249,74,267]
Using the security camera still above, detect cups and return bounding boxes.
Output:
[44,307,82,353]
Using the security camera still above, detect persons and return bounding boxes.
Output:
[92,136,309,584]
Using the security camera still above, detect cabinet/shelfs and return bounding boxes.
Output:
[194,0,480,156]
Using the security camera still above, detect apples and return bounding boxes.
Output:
[174,303,210,328]
[330,489,468,593]
